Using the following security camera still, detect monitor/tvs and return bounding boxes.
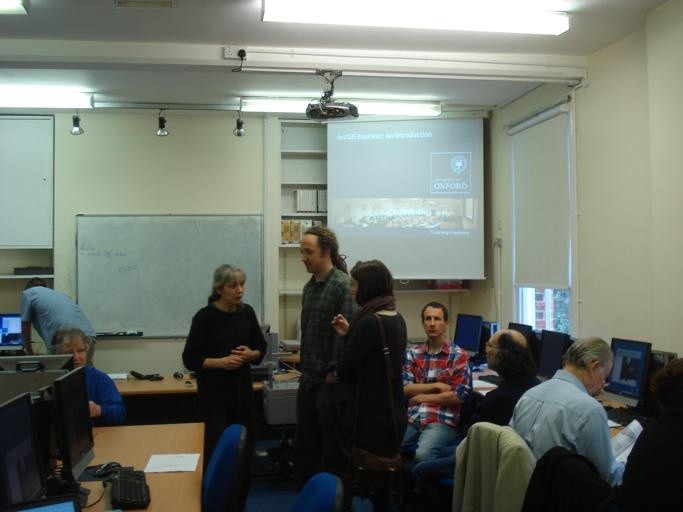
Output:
[0,392,47,505]
[536,329,571,378]
[0,494,81,512]
[508,322,532,348]
[603,338,652,399]
[453,314,483,352]
[41,366,97,481]
[0,354,74,372]
[0,313,23,345]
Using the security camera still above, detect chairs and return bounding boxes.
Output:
[203,421,247,506]
[521,446,614,512]
[449,422,534,510]
[289,472,344,512]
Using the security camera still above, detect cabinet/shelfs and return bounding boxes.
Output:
[277,119,471,294]
[0,115,55,281]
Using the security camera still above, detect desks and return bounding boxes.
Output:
[105,369,300,396]
[459,366,629,453]
[38,424,205,511]
[278,346,303,365]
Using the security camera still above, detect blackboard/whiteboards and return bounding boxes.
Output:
[74,213,265,340]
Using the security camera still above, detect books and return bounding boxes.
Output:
[292,189,328,213]
[281,219,323,245]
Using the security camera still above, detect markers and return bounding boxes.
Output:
[117,331,144,336]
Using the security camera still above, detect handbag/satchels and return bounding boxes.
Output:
[347,314,404,474]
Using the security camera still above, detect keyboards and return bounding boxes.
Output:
[602,406,649,429]
[111,467,151,510]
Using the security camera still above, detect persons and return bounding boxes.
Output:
[411,329,541,512]
[296,227,357,511]
[623,357,683,512]
[182,264,268,512]
[325,260,407,512]
[683,512,683,512]
[399,302,472,464]
[52,328,126,426]
[509,336,625,489]
[19,278,97,369]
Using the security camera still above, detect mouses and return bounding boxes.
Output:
[94,462,121,477]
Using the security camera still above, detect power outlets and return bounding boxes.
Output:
[223,45,248,60]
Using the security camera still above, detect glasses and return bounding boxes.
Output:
[596,367,611,387]
[485,341,499,349]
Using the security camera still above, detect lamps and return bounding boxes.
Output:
[71,114,85,135]
[156,109,169,136]
[233,112,247,137]
[90,93,242,109]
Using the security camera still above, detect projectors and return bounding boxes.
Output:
[306,102,358,121]
